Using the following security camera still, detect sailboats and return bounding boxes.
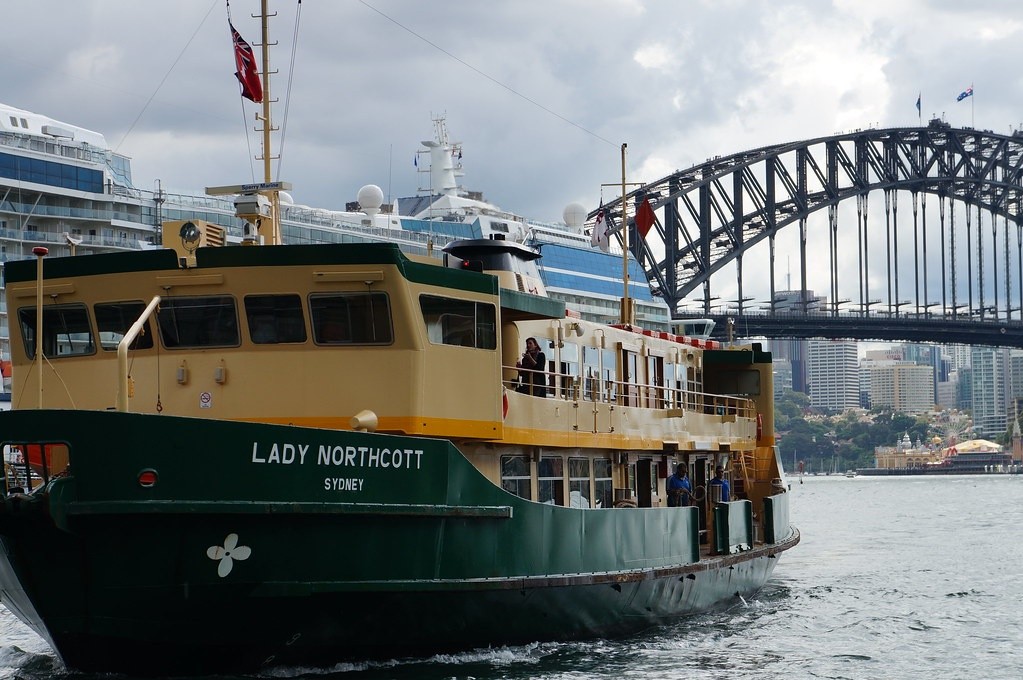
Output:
[784,449,857,478]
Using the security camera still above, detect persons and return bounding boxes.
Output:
[666,464,691,507]
[710,466,729,502]
[515,338,546,398]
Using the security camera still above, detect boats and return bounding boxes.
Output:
[1,0,799,680]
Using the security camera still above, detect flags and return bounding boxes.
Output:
[591,202,610,252]
[231,25,262,103]
[957,87,973,102]
[916,98,920,117]
[634,199,654,241]
